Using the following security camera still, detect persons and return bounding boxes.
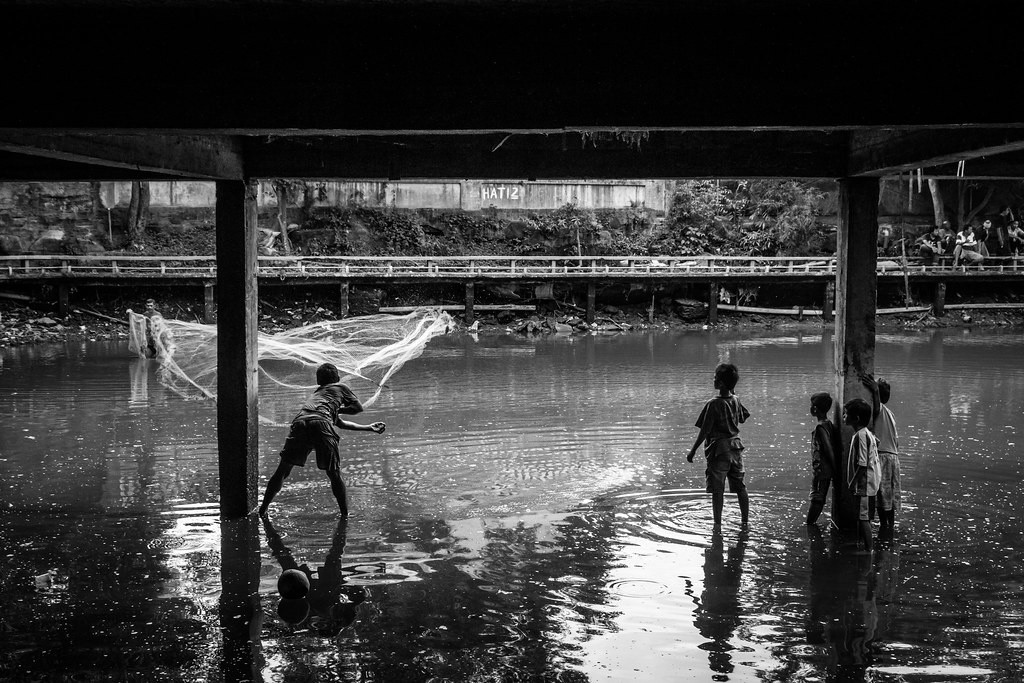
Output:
[686,528,748,677]
[862,373,901,525]
[843,399,883,548]
[262,516,371,639]
[126,299,163,356]
[260,363,386,517]
[807,393,836,523]
[919,204,1024,272]
[686,364,750,522]
